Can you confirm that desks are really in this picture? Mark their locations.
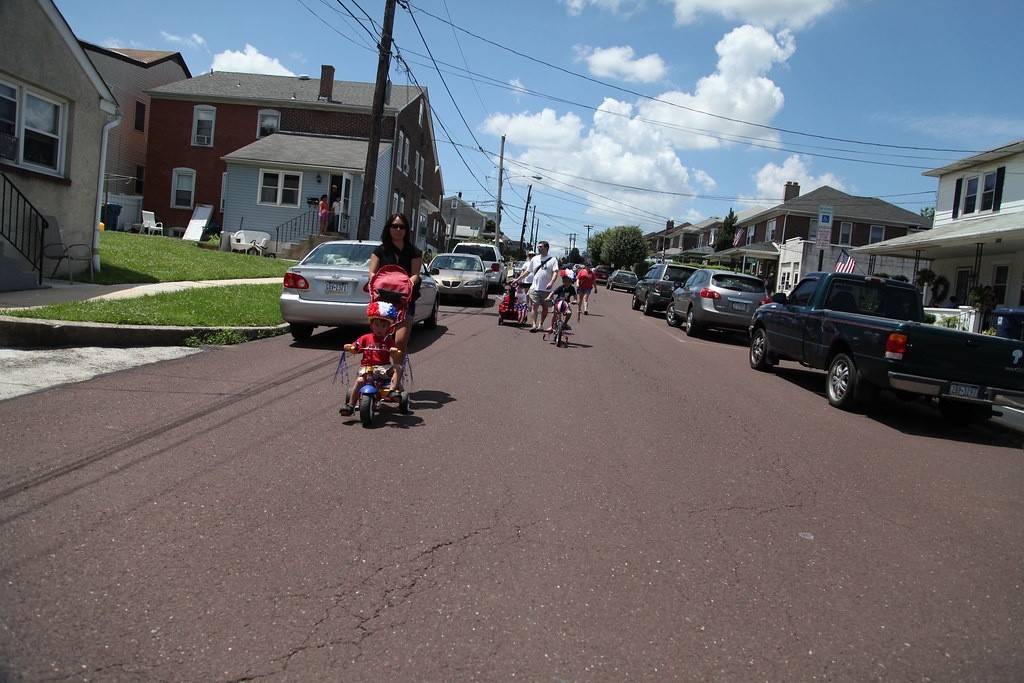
[169,227,187,237]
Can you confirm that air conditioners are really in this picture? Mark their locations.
[194,134,211,145]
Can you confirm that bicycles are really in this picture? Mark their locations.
[543,298,576,347]
[247,240,276,258]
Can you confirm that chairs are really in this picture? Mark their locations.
[830,291,857,314]
[138,210,163,237]
[31,213,94,285]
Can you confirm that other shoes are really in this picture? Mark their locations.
[339,403,355,414]
[584,310,588,315]
[529,326,537,333]
[538,322,544,331]
[385,390,402,403]
[562,324,571,330]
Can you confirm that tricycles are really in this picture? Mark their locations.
[342,266,411,423]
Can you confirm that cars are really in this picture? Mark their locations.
[279,240,440,341]
[424,253,492,305]
[606,270,638,294]
[562,263,579,272]
[513,261,525,278]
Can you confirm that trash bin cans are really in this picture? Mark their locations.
[101,202,122,231]
[992,307,1024,341]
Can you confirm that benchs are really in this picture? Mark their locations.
[230,229,271,256]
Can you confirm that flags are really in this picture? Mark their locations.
[833,250,856,274]
[731,225,744,247]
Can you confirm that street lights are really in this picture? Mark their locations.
[494,175,542,248]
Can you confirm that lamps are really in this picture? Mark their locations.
[316,174,321,181]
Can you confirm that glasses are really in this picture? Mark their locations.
[537,246,543,248]
[392,223,409,229]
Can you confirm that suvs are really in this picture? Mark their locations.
[632,262,698,315]
[592,265,612,283]
[452,243,507,290]
[664,268,767,337]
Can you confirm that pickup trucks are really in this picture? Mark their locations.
[748,270,1024,423]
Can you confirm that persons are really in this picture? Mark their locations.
[369,213,422,390]
[945,296,957,309]
[318,184,341,235]
[543,269,578,332]
[513,284,527,323]
[519,250,537,302]
[336,301,402,416]
[575,261,598,314]
[510,241,559,333]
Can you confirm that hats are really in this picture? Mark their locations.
[366,300,398,326]
[560,268,577,284]
